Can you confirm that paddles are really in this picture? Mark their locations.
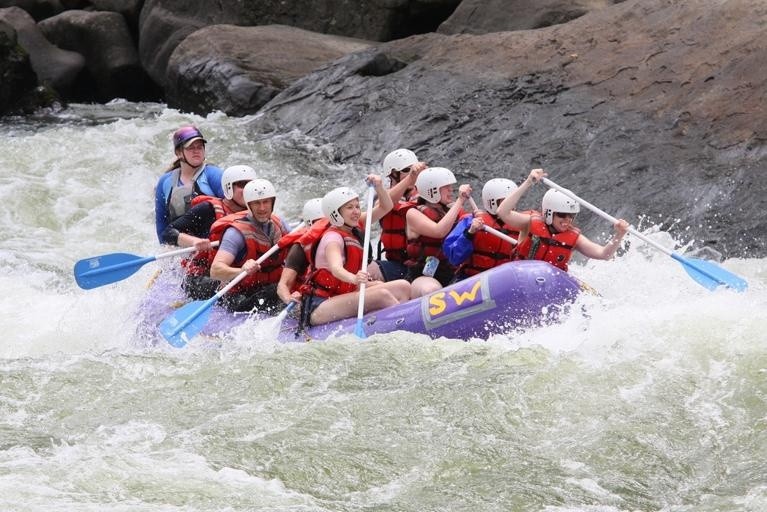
[533,174,749,294]
[73,239,220,291]
[354,180,374,338]
[162,218,305,349]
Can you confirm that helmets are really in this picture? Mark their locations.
[542,187,580,226]
[173,125,204,148]
[383,149,419,177]
[482,178,519,215]
[415,166,458,204]
[221,164,276,217]
[303,186,360,228]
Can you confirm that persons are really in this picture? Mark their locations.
[405,163,471,288]
[444,178,529,287]
[371,148,427,278]
[160,166,255,298]
[275,197,324,304]
[208,178,291,314]
[152,125,225,243]
[298,174,410,328]
[497,168,632,272]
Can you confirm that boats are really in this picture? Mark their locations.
[133,222,607,369]
